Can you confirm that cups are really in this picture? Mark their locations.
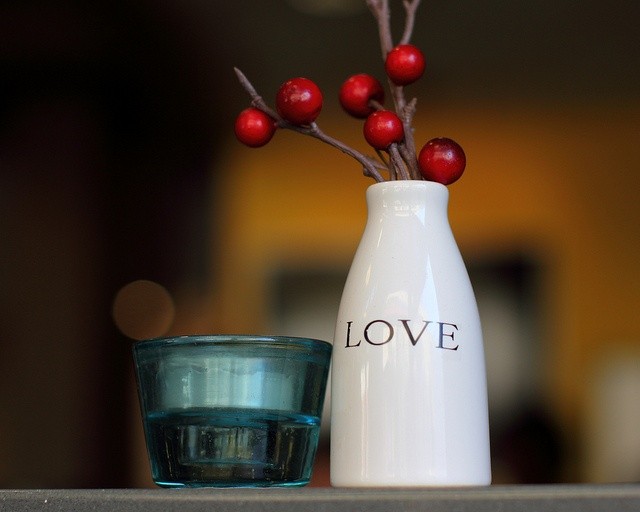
[135,336,332,486]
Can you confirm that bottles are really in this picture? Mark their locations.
[330,178,492,484]
